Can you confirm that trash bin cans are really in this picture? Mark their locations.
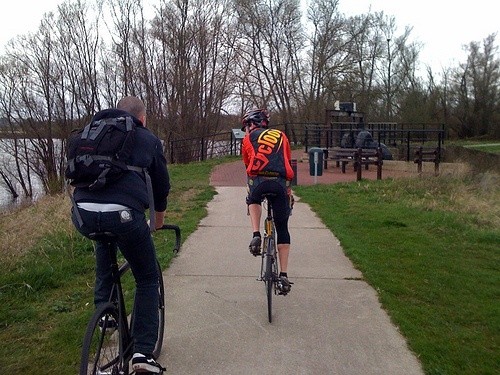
[308,148,324,177]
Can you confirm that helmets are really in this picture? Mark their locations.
[241,108,269,131]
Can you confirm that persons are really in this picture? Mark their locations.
[241,108,295,288]
[66,96,171,375]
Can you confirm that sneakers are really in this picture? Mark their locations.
[99,318,118,333]
[132,353,162,374]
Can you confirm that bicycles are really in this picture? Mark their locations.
[79,224,182,375]
[246,193,295,322]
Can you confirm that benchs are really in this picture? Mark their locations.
[324,148,377,172]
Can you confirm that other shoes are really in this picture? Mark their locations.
[279,276,290,293]
[250,237,260,255]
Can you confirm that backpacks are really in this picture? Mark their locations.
[68,116,148,191]
